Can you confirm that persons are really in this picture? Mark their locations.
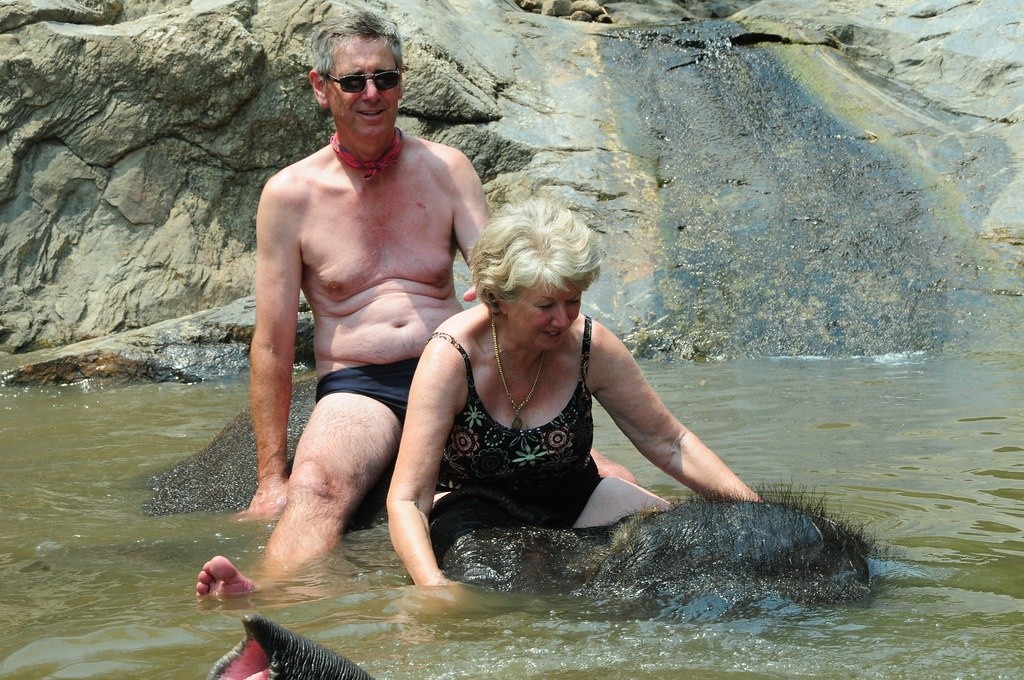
[386,196,766,590]
[193,11,493,606]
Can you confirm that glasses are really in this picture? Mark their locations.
[321,67,400,93]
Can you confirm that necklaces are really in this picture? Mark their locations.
[491,314,545,430]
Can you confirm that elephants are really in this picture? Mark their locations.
[141,377,897,680]
